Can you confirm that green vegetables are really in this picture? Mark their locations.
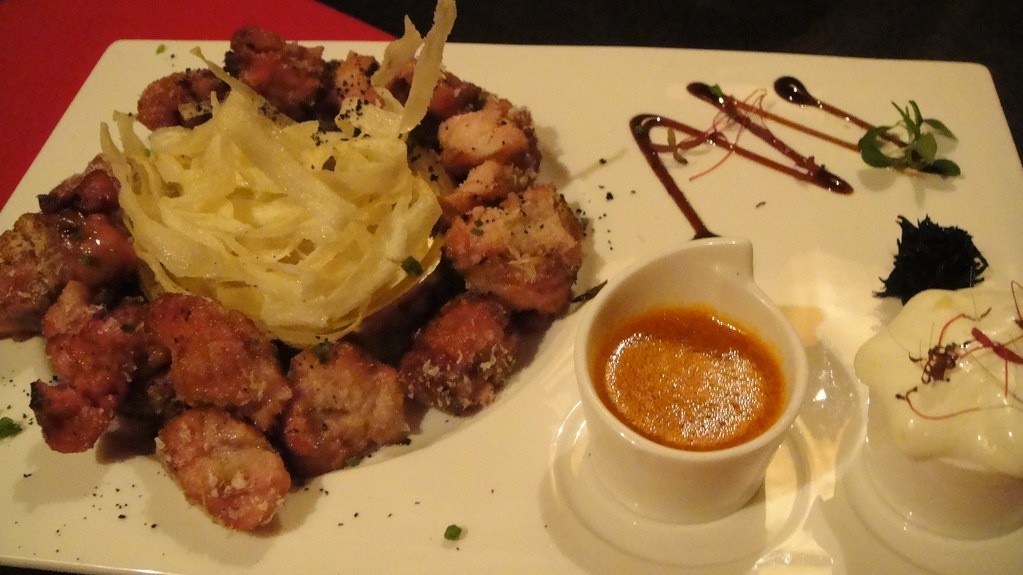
[859,97,962,178]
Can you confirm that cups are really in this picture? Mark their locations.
[862,393,1023,540]
[577,238,811,526]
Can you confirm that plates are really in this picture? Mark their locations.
[2,37,1023,574]
[541,399,821,569]
